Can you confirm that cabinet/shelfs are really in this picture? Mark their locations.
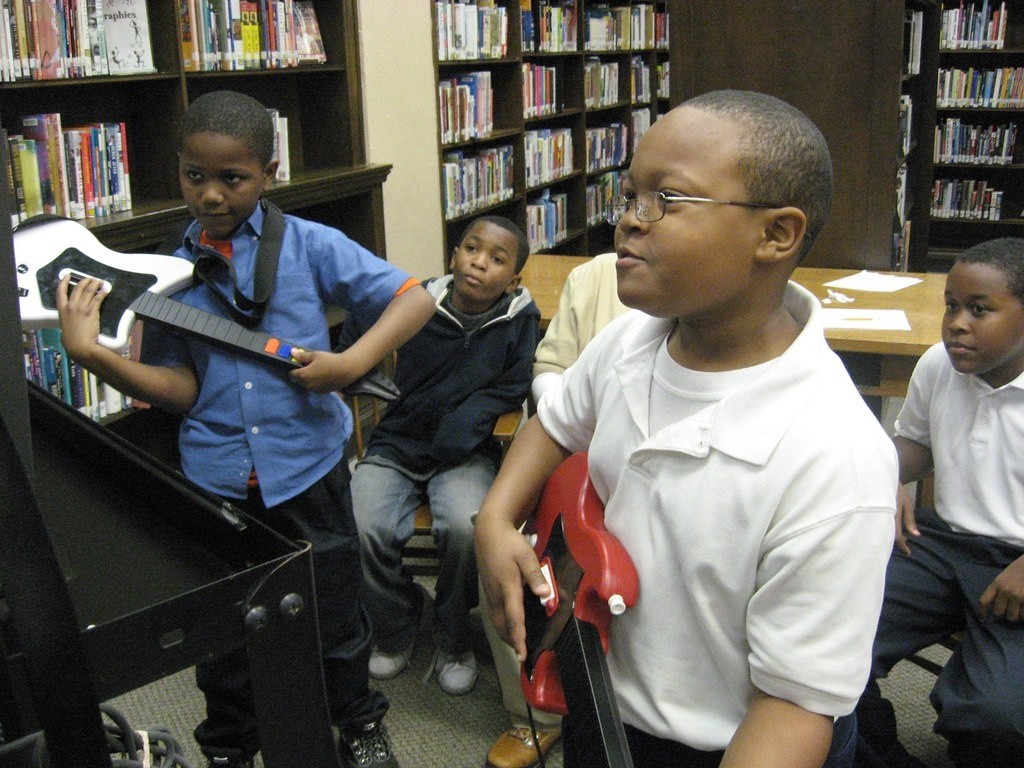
[431,0,674,276]
[892,0,1023,274]
[1,0,393,464]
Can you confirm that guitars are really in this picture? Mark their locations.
[520,451,641,767]
[12,213,400,403]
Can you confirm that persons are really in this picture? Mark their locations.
[473,87,902,767]
[346,214,542,699]
[856,234,1024,766]
[54,89,437,767]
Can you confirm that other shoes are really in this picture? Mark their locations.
[338,721,400,768]
[201,745,254,768]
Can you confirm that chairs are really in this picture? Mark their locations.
[351,391,523,576]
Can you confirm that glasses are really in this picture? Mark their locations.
[601,191,811,239]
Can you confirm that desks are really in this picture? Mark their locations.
[521,253,948,509]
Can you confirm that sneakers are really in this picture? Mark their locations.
[438,650,477,695]
[368,636,414,679]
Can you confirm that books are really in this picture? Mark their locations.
[436,2,670,268]
[0,0,392,429]
[889,2,1024,268]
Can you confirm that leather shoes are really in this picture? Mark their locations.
[487,726,561,768]
